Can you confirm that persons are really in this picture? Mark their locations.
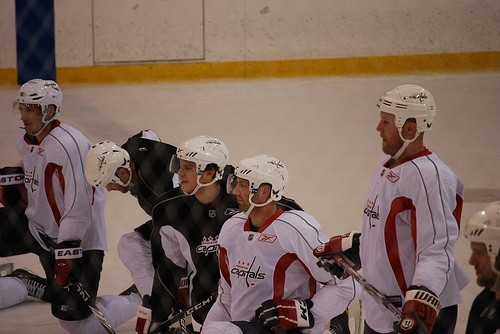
[0,78,141,334]
[464,200,500,334]
[312,82,471,334]
[85,128,364,334]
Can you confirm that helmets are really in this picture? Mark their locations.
[464,201,500,274]
[85,140,132,190]
[12,79,63,123]
[377,84,436,142]
[227,154,289,206]
[169,135,228,186]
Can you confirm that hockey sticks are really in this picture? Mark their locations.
[37,224,117,334]
[178,305,187,334]
[150,292,217,334]
[333,254,402,321]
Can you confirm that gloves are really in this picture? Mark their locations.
[135,295,169,334]
[0,166,28,209]
[313,232,362,280]
[255,299,315,332]
[393,285,441,334]
[51,240,84,292]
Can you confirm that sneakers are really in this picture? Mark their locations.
[12,269,50,302]
[327,298,362,334]
